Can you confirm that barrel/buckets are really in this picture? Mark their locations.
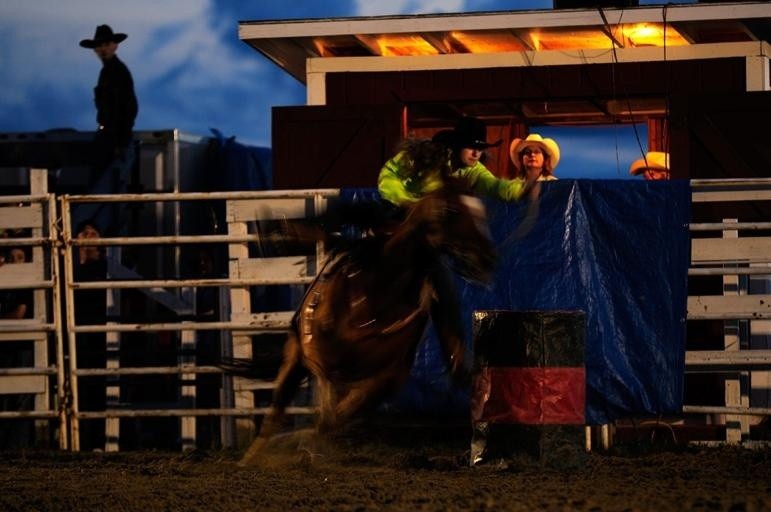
[472,308,587,471]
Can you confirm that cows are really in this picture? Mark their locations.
[198,163,497,467]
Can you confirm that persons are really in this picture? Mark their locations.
[254,117,541,371]
[0,248,28,319]
[73,222,103,281]
[78,24,139,193]
[506,131,560,181]
[629,150,670,180]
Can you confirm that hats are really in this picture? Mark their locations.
[440,117,503,150]
[630,151,672,177]
[80,24,127,48]
[509,133,560,173]
